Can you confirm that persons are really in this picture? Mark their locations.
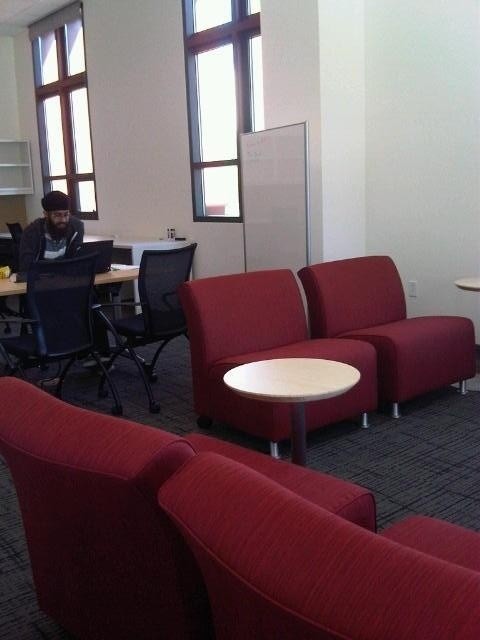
[18,191,114,376]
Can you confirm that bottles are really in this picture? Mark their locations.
[167,228,175,241]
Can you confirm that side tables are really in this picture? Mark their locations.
[224,357,361,467]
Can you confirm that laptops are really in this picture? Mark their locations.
[76,240,113,275]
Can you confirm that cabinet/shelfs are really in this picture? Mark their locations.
[0,140,36,195]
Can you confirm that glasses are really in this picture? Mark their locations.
[52,213,70,218]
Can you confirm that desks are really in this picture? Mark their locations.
[0,261,180,366]
[0,232,190,317]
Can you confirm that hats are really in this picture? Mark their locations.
[41,191,73,210]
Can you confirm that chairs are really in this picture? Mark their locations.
[0,251,123,416]
[180,268,378,458]
[92,243,197,412]
[0,374,377,640]
[5,220,23,244]
[297,255,476,419]
[155,450,478,639]
